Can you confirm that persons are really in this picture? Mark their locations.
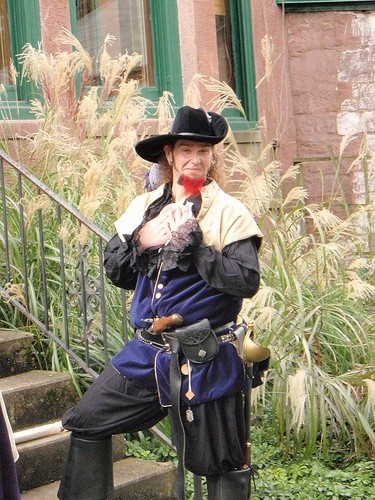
[57,106,270,500]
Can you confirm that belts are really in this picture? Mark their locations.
[138,326,236,500]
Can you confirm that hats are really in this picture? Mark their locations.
[135,106,228,163]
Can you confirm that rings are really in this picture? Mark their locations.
[171,231,176,235]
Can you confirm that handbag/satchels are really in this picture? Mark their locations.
[175,318,219,363]
